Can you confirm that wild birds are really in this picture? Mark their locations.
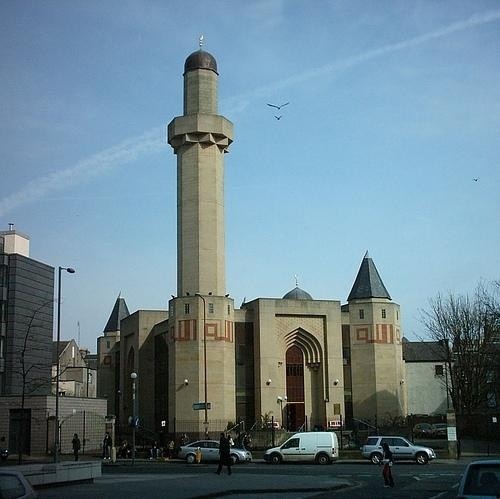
[274,116,281,120]
[267,102,290,110]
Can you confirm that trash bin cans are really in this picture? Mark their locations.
[147,448,164,457]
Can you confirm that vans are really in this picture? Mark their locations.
[263,432,339,465]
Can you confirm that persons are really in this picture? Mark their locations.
[152,433,190,459]
[228,433,253,453]
[214,433,232,476]
[196,446,203,464]
[381,442,395,488]
[72,434,80,461]
[119,439,132,462]
[103,432,112,459]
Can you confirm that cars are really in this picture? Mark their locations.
[413,423,446,439]
[266,421,281,431]
[457,457,500,499]
[0,471,36,499]
[178,440,252,464]
[360,436,436,465]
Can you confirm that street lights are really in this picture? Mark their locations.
[195,293,209,446]
[130,372,137,466]
[54,267,75,463]
[487,416,497,453]
